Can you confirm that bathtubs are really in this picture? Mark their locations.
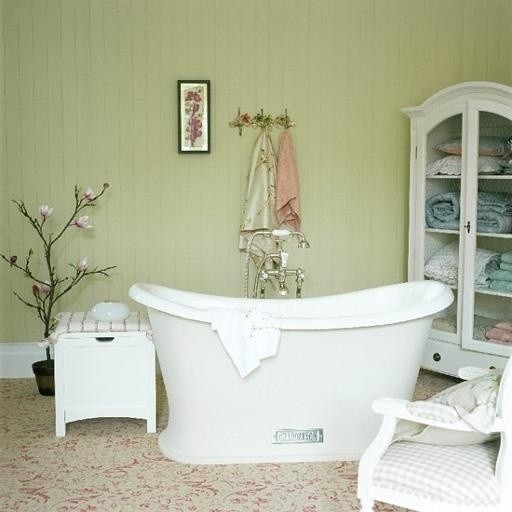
[129,279,455,466]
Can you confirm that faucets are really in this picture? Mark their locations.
[277,272,288,295]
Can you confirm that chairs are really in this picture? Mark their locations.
[357,355,512,512]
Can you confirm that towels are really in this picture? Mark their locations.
[276,129,302,234]
[484,319,512,347]
[239,128,276,259]
[488,252,512,294]
[208,305,281,379]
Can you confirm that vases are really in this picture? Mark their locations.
[31,358,58,397]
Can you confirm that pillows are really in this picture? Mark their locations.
[432,136,512,159]
[388,368,504,446]
[426,154,505,176]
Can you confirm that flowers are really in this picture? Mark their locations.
[184,89,204,146]
[0,180,118,366]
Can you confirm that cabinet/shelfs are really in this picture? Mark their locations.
[400,80,512,379]
[44,312,160,438]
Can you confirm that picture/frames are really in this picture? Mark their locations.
[177,80,210,154]
